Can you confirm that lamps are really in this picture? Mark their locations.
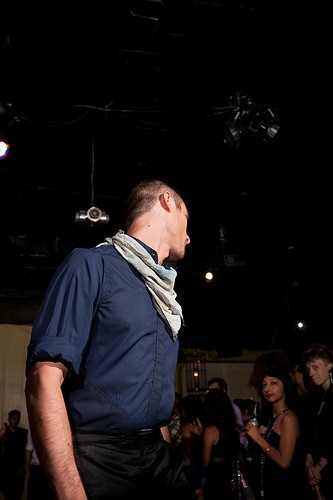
[213,91,281,152]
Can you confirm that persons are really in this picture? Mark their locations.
[24,429,35,479]
[159,342,333,500]
[25,176,200,500]
[0,410,29,500]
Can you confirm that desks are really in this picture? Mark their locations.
[175,360,263,405]
